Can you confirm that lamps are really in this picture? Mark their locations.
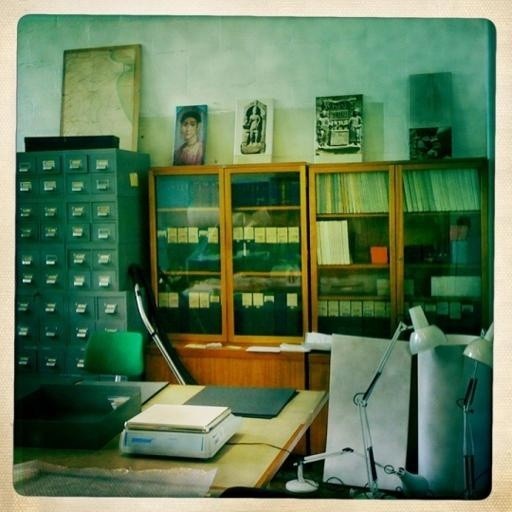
[285,306,493,497]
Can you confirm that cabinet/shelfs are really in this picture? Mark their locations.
[308,158,489,453]
[16,150,151,370]
[148,162,310,455]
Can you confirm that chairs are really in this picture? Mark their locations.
[84,330,145,382]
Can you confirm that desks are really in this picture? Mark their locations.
[14,384,329,498]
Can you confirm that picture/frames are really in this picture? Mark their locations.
[61,46,139,151]
[313,94,358,158]
[233,98,273,164]
[176,106,205,162]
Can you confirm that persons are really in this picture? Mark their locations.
[246,106,261,146]
[173,112,202,164]
[317,105,361,146]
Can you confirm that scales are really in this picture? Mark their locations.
[119,404,240,461]
